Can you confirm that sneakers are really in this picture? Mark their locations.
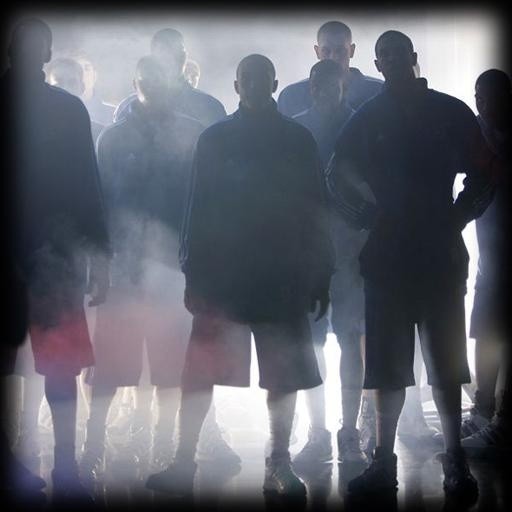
[2,403,241,495]
[293,425,334,464]
[458,407,489,436]
[358,412,378,455]
[348,454,398,495]
[262,455,306,498]
[459,422,508,451]
[336,428,369,465]
[436,448,479,504]
[397,417,439,438]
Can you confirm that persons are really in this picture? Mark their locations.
[1,18,512,499]
